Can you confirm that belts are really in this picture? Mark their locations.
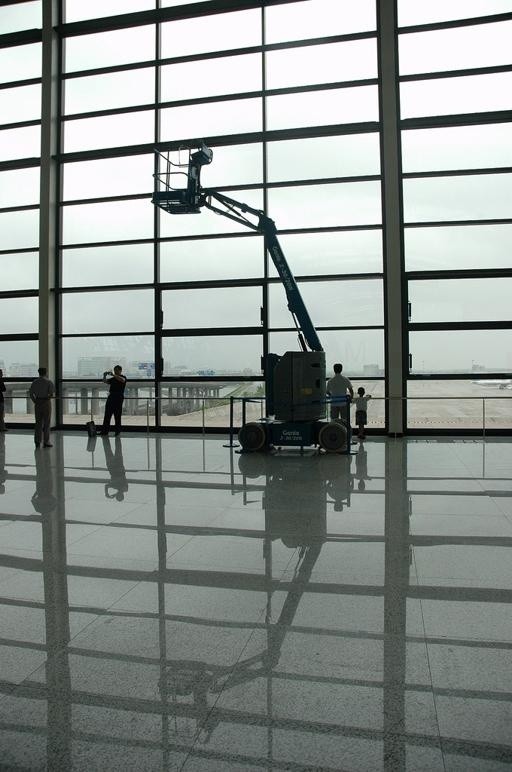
[36,398,50,400]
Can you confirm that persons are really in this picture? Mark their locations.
[326,364,357,444]
[97,365,127,435]
[348,387,371,439]
[30,367,54,447]
[0,369,8,432]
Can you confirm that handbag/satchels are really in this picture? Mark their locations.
[86,421,96,437]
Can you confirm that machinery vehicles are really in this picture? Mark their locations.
[154,136,360,458]
[148,454,355,743]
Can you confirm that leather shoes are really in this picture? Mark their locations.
[96,430,120,436]
[35,442,53,447]
